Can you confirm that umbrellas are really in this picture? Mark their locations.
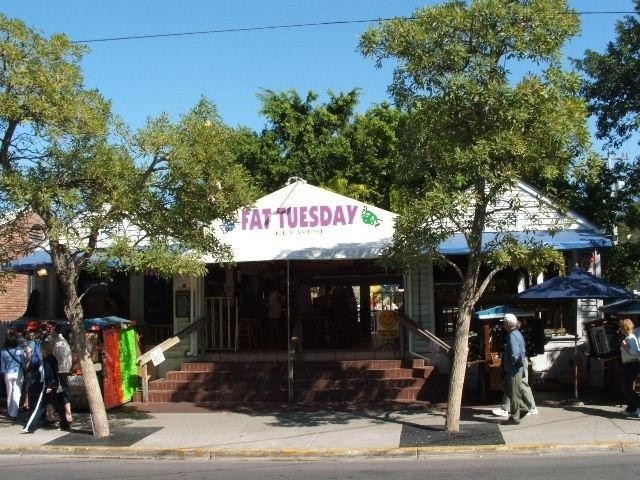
[514,265,637,399]
[597,290,640,319]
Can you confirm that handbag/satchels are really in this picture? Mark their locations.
[515,357,529,367]
[619,347,640,363]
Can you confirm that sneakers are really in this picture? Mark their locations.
[491,406,538,425]
[622,409,640,418]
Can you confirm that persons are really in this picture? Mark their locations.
[0,319,73,433]
[490,319,539,418]
[498,311,526,424]
[618,317,640,418]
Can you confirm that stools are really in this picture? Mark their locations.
[239,319,264,346]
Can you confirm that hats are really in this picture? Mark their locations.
[29,322,37,329]
[500,313,516,323]
